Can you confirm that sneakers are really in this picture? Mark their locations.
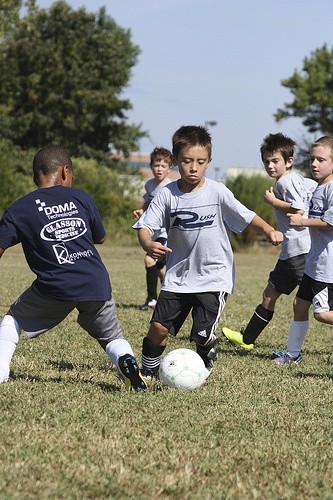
[139,295,158,311]
[272,353,303,365]
[141,372,157,385]
[221,326,254,351]
[117,353,148,393]
[202,358,214,379]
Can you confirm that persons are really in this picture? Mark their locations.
[131,125,284,380]
[133,146,173,310]
[221,133,319,349]
[0,145,148,393]
[271,136,333,365]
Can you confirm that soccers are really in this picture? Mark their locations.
[157,348,209,389]
